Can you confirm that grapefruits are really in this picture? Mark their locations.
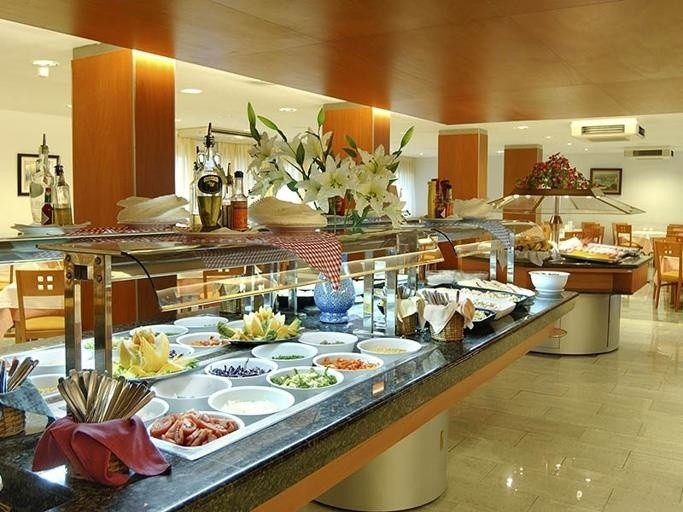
[119,332,169,372]
[243,310,285,336]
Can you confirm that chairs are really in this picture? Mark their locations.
[15,270,64,343]
[563,221,683,312]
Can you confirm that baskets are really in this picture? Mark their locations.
[423,293,466,342]
[64,441,130,480]
[379,287,418,335]
[0,403,26,439]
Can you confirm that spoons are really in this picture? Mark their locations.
[57,368,156,423]
[382,283,418,298]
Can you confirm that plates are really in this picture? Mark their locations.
[263,222,326,232]
[182,231,261,245]
[423,217,464,223]
[117,220,179,229]
[324,214,352,221]
[13,226,87,235]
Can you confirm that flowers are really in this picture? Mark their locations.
[522,151,589,190]
[246,101,415,235]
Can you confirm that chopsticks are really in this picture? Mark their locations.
[422,289,461,306]
[1,356,39,392]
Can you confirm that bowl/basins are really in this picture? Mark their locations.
[296,331,358,355]
[223,319,246,331]
[357,337,423,364]
[251,342,319,368]
[203,357,278,385]
[265,366,344,400]
[174,333,230,352]
[174,316,228,333]
[313,352,384,379]
[167,342,196,358]
[120,366,192,382]
[229,331,300,348]
[150,373,232,414]
[147,411,246,447]
[528,271,571,295]
[134,397,169,425]
[128,324,190,342]
[208,386,296,425]
[528,295,564,315]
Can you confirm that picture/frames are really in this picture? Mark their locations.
[589,167,623,195]
[17,153,59,196]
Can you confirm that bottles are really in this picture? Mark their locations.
[191,122,249,232]
[427,178,454,218]
[29,135,74,225]
[329,196,347,214]
